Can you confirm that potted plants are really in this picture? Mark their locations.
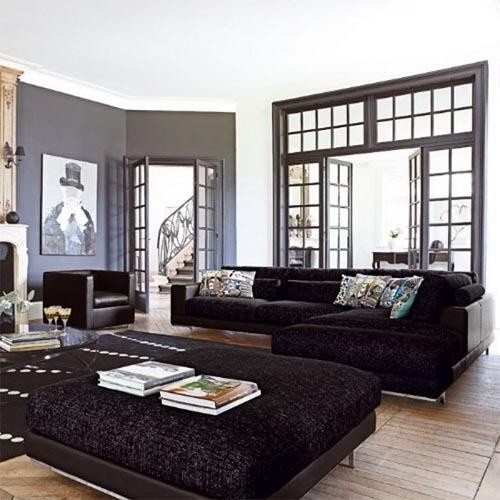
[0,289,35,333]
[288,214,312,248]
[387,228,401,252]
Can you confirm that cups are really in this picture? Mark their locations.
[44,308,55,335]
[59,308,71,336]
[51,306,61,333]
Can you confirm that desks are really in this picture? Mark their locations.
[372,252,455,272]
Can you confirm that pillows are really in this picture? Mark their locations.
[407,273,473,323]
[343,274,392,309]
[333,274,355,305]
[390,278,424,319]
[252,278,282,300]
[286,279,341,302]
[380,278,408,308]
[199,269,221,296]
[216,269,256,299]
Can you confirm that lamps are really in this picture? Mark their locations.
[6,146,26,169]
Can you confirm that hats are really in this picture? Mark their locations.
[60,163,84,191]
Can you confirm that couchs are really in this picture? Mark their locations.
[24,344,383,500]
[43,269,136,330]
[171,265,478,335]
[271,273,496,405]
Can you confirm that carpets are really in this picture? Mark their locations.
[0,330,272,463]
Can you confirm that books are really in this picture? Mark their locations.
[158,374,263,416]
[0,330,62,353]
[95,359,196,397]
